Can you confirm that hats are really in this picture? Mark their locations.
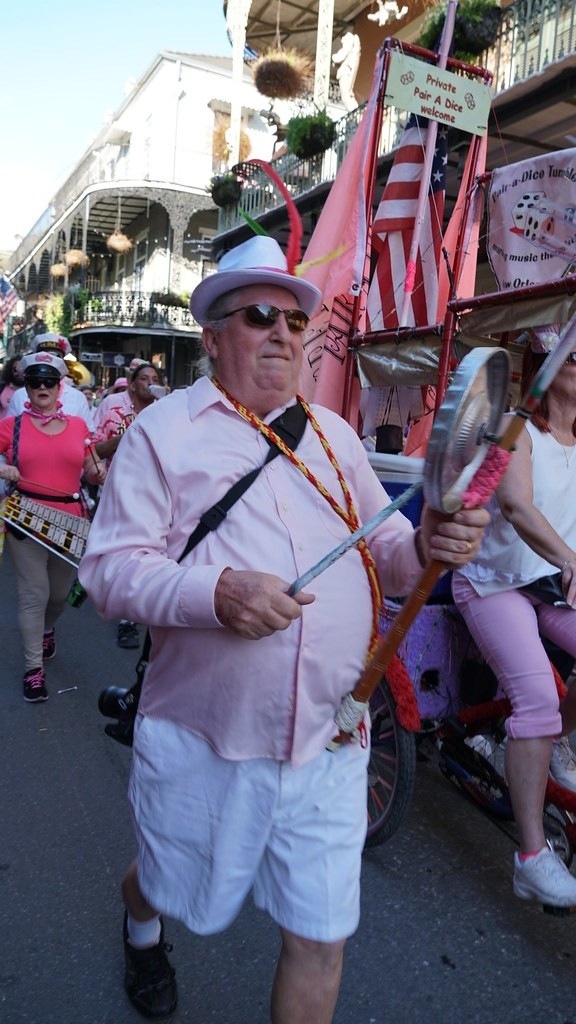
[16,351,69,382]
[190,236,322,325]
[109,377,128,395]
[31,334,72,357]
[124,358,146,374]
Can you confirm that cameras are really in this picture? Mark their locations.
[98,685,139,748]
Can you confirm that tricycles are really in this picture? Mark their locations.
[352,276,575,919]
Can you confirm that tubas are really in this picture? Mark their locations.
[63,360,91,388]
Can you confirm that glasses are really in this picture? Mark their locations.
[563,352,576,367]
[13,367,17,372]
[27,377,59,389]
[217,304,309,331]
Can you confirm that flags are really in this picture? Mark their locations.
[287,37,489,459]
[0,277,20,326]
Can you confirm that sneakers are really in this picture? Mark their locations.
[117,621,141,648]
[513,838,576,906]
[43,627,57,660]
[22,667,49,701]
[123,912,179,1020]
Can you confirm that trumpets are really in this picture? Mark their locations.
[116,404,137,436]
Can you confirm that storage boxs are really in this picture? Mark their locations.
[367,451,453,599]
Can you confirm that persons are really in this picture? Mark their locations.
[95,358,171,650]
[331,32,361,123]
[0,332,107,703]
[451,323,576,909]
[76,236,490,1023]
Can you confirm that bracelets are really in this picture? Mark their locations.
[560,557,576,572]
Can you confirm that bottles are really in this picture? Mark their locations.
[148,384,166,400]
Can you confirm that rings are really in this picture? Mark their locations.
[466,542,472,553]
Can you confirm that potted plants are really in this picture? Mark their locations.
[284,105,338,160]
[41,286,90,341]
[151,286,190,309]
[414,0,507,63]
[205,172,243,207]
